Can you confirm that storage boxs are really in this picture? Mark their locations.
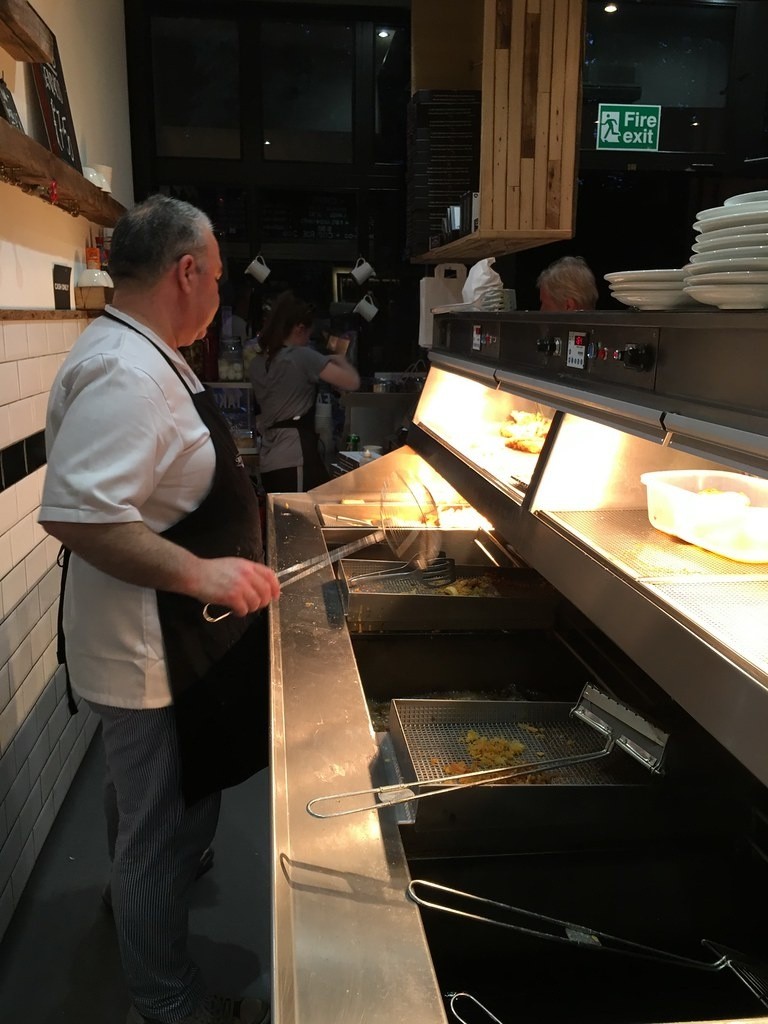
[640,469,768,565]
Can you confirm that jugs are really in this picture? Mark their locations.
[350,257,377,286]
[352,294,379,322]
[244,255,271,283]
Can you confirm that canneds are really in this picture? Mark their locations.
[345,435,359,451]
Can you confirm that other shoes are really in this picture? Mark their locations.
[100,847,215,908]
[144,992,270,1024]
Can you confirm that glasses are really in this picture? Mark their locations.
[296,303,318,326]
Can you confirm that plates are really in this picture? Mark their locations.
[603,269,695,310]
[683,190,768,310]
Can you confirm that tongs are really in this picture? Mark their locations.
[429,289,516,313]
[348,550,457,587]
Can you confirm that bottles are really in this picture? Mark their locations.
[371,375,427,393]
[88,227,111,273]
[218,335,245,382]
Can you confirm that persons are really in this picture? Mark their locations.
[250,290,361,493]
[37,196,279,1022]
